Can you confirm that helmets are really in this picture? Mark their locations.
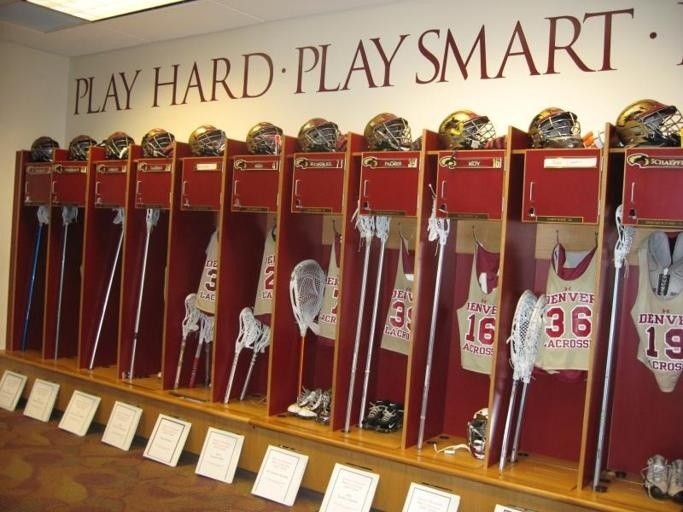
[67,136,98,160]
[438,109,497,150]
[246,122,283,154]
[364,113,416,152]
[616,99,683,148]
[467,407,488,460]
[142,128,175,157]
[105,132,135,159]
[297,118,344,152]
[189,125,225,155]
[528,107,584,148]
[30,137,58,162]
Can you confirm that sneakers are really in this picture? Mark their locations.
[287,385,332,426]
[362,400,404,433]
[640,455,682,505]
